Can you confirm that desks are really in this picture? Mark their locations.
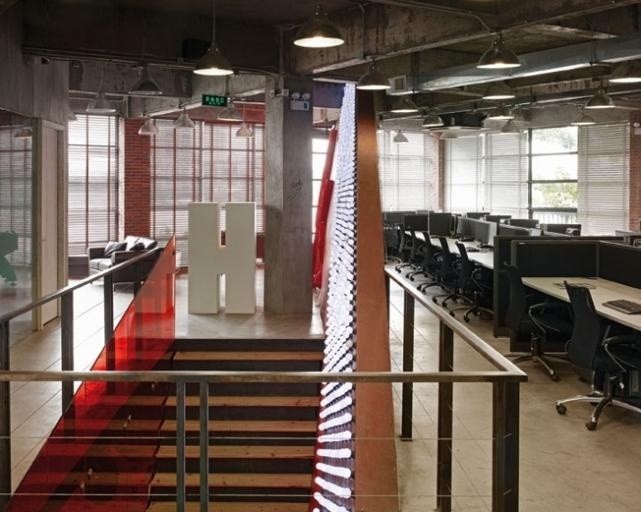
[523,276,641,383]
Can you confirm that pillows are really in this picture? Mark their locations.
[127,241,145,253]
[102,238,124,261]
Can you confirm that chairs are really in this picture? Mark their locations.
[555,279,641,431]
[502,261,576,381]
[382,209,497,325]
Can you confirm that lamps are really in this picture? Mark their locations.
[291,2,346,50]
[472,30,526,70]
[191,0,235,79]
[355,55,391,90]
[137,101,254,139]
[481,79,516,103]
[126,62,168,97]
[85,63,120,115]
[584,28,617,111]
[392,106,523,143]
[569,108,597,126]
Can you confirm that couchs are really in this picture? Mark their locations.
[86,232,164,291]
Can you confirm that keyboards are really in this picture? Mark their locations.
[602,299,641,314]
